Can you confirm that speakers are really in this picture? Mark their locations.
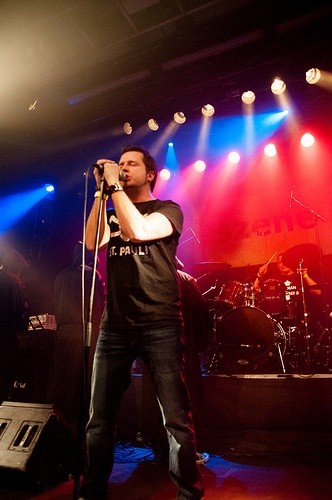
[0,401,87,492]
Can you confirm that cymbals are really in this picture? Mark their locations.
[194,261,232,272]
[280,243,323,270]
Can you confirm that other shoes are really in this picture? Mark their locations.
[196,452,210,464]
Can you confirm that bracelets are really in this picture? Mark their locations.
[106,179,124,195]
[91,191,110,200]
[301,272,309,279]
[93,184,110,194]
[257,274,264,281]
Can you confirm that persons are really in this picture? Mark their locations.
[80,140,205,500]
[53,241,104,413]
[1,249,32,332]
[252,248,322,336]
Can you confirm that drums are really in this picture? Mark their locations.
[214,306,287,373]
[215,280,243,308]
[260,279,285,315]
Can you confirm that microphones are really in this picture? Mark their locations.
[290,192,293,208]
[93,163,126,182]
[189,228,201,244]
